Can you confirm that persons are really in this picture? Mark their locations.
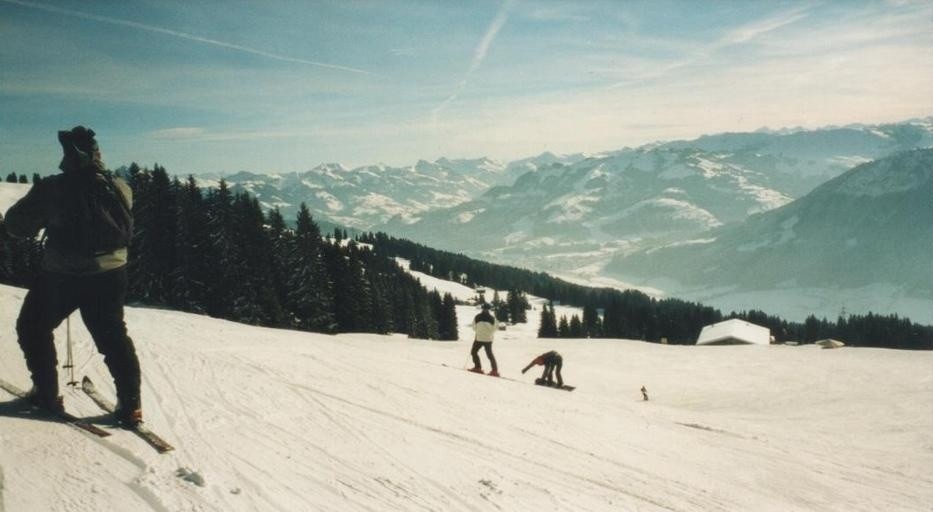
[522,351,563,387]
[471,305,498,377]
[4,125,141,427]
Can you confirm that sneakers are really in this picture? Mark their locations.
[113,404,144,427]
[8,390,65,414]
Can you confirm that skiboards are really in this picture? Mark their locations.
[0,375,176,454]
[441,364,525,384]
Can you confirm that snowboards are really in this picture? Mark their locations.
[535,378,576,391]
